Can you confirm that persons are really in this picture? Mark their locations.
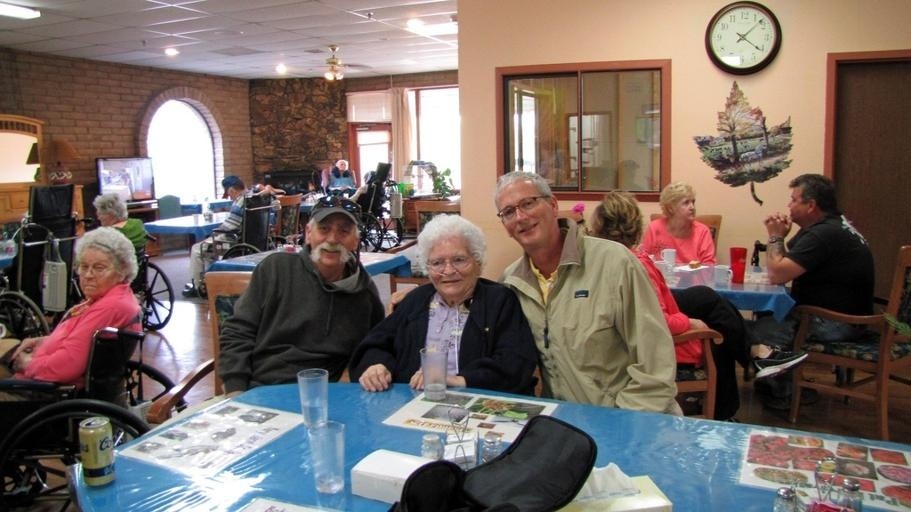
[211,194,386,399]
[752,172,878,418]
[348,213,538,397]
[348,170,379,205]
[327,159,358,198]
[591,186,808,425]
[635,179,717,267]
[0,224,144,401]
[105,164,149,192]
[670,262,715,291]
[493,168,685,418]
[180,175,255,299]
[91,191,149,247]
[536,138,656,190]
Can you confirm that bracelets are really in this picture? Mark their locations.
[767,235,785,243]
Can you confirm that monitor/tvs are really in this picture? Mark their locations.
[95,157,158,208]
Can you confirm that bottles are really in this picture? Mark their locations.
[771,487,796,512]
[421,434,442,458]
[480,432,502,462]
[835,478,864,511]
[201,196,214,221]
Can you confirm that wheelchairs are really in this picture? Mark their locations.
[0,304,185,509]
[200,192,276,301]
[327,161,406,256]
[0,181,94,341]
[69,229,176,334]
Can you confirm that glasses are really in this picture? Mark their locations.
[496,195,550,218]
[318,196,360,213]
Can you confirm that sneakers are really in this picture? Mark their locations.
[766,385,818,409]
[183,283,207,297]
[752,347,808,379]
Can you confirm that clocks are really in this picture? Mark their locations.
[706,0,782,76]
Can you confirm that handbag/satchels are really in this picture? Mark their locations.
[42,240,67,312]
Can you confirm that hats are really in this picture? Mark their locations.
[310,196,360,225]
[221,176,240,199]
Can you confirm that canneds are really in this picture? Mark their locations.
[78,418,113,487]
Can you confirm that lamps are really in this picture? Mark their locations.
[26,143,41,181]
[41,141,81,185]
[322,44,344,82]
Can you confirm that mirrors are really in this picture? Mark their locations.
[0,114,45,183]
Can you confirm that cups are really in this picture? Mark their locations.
[304,192,324,203]
[730,247,747,284]
[420,345,449,401]
[713,264,733,284]
[297,368,329,429]
[308,421,344,493]
[343,193,349,198]
[661,248,676,266]
[654,261,667,271]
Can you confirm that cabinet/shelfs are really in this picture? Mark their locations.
[0,184,84,236]
